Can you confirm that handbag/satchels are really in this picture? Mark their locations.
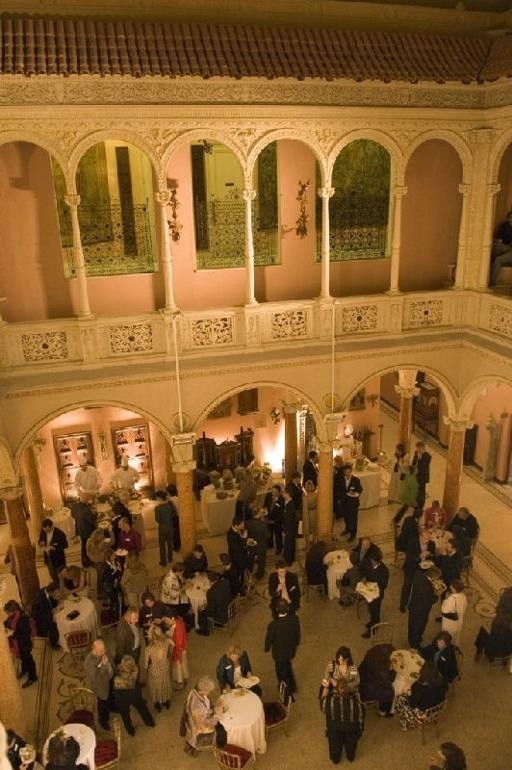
[319,672,334,699]
[441,613,458,621]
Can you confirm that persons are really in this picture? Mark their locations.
[1,431,512,770]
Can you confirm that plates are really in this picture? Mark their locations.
[248,675,260,685]
[247,541,258,546]
[21,749,37,764]
[346,492,359,497]
[115,548,128,557]
[418,560,434,570]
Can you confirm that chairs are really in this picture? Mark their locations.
[94,716,122,770]
[58,567,98,600]
[448,646,465,699]
[457,555,472,588]
[64,630,92,657]
[406,700,447,746]
[263,680,291,740]
[299,563,326,603]
[345,585,370,618]
[370,622,395,648]
[180,709,216,755]
[213,743,255,770]
[210,592,239,639]
[357,686,381,717]
[101,593,123,634]
[66,687,97,727]
[393,524,406,565]
[240,569,252,613]
[468,523,480,555]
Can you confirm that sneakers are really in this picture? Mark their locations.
[17,670,25,679]
[22,678,37,687]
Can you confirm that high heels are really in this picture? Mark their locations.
[163,702,170,709]
[155,704,161,713]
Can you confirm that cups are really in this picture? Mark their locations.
[350,487,354,495]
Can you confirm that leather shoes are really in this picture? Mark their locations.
[347,536,355,542]
[340,531,348,536]
[379,713,394,719]
[196,629,209,636]
[101,722,110,731]
[366,622,373,627]
[362,629,375,638]
[435,617,442,622]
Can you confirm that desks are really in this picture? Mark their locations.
[182,570,217,629]
[214,688,268,753]
[52,597,102,652]
[426,529,458,557]
[200,478,280,537]
[389,650,426,713]
[42,723,96,770]
[352,464,381,510]
[324,550,353,599]
[42,503,75,541]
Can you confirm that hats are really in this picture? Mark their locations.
[345,424,353,435]
[121,455,128,466]
[79,459,87,465]
